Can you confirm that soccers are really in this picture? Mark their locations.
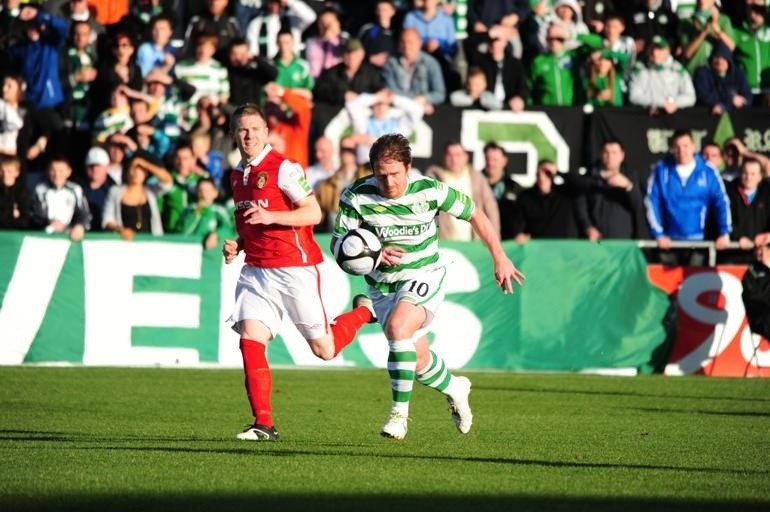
[334,228,384,275]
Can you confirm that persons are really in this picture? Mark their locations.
[1,1,770,240]
[330,132,526,441]
[741,232,770,343]
[221,103,379,442]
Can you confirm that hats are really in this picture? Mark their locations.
[86,147,110,166]
[344,38,361,50]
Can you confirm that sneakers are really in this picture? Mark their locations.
[381,413,408,440]
[446,377,472,434]
[235,423,280,441]
[353,294,379,323]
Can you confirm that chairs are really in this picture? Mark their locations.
[741,261,770,378]
